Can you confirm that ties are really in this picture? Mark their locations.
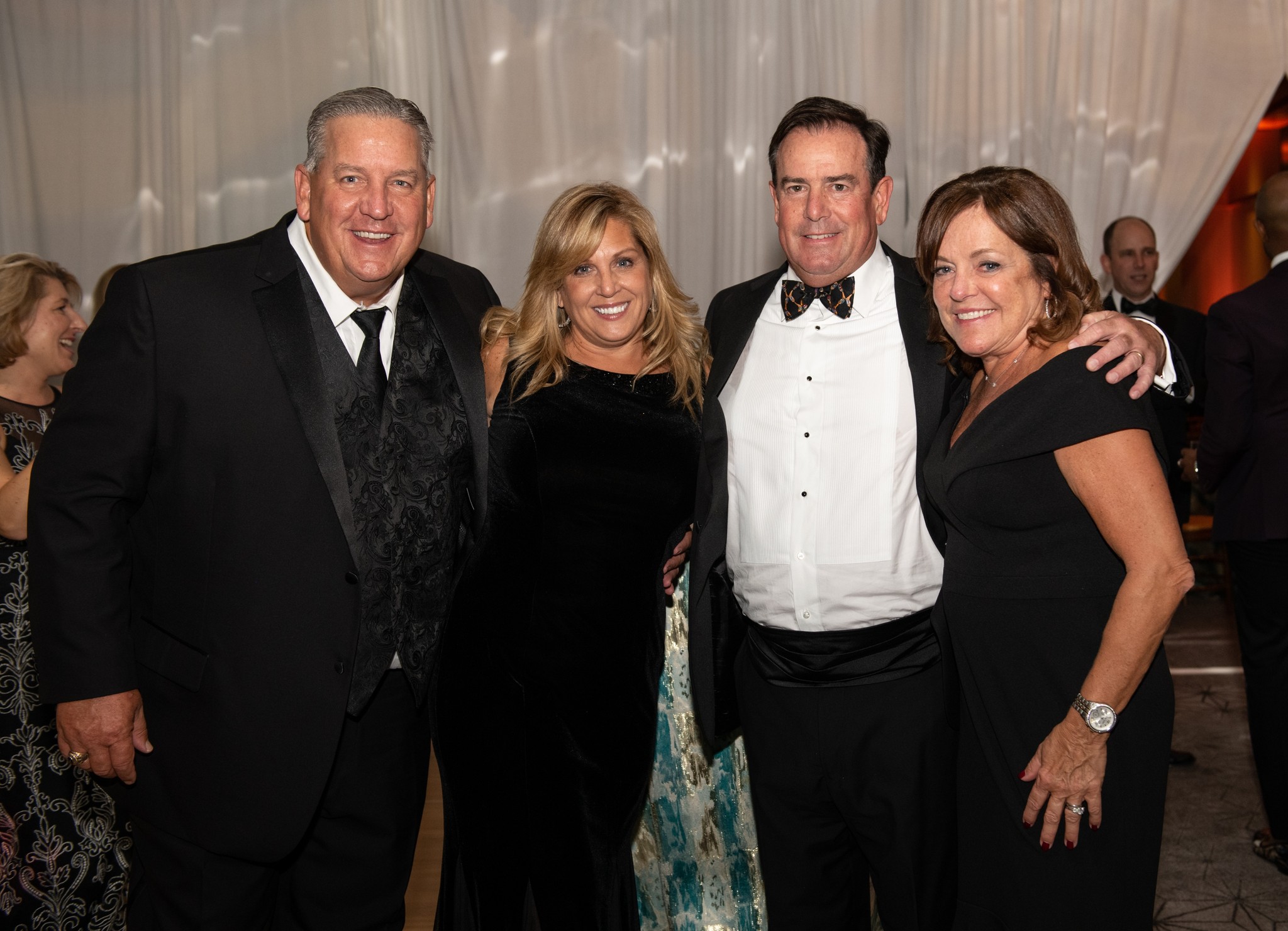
[350,306,391,405]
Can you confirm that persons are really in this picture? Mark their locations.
[916,168,1199,931]
[0,254,134,931]
[685,98,1197,931]
[27,84,695,931]
[430,184,719,930]
[1204,170,1288,877]
[1102,215,1209,553]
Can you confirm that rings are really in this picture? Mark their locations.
[1065,802,1085,815]
[1127,351,1144,367]
[67,750,90,765]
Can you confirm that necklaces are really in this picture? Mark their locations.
[984,342,1031,388]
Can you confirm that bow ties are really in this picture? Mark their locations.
[781,275,856,319]
[1121,297,1156,316]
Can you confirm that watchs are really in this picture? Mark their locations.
[1072,692,1119,734]
